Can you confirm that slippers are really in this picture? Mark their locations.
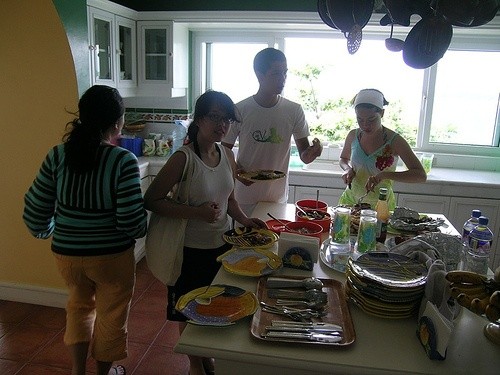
[111,365,126,375]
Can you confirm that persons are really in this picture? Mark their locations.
[143,90,270,375]
[220,47,323,232]
[22,85,149,375]
[337,89,427,211]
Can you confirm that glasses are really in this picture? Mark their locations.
[202,114,234,124]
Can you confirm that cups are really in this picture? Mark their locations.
[353,217,378,261]
[361,210,377,218]
[330,207,352,255]
[420,153,434,175]
[118,132,170,159]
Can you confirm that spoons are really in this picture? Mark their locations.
[267,276,323,289]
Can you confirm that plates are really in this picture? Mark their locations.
[222,226,280,248]
[174,284,260,327]
[319,236,390,274]
[344,250,429,320]
[237,169,286,183]
[265,219,291,235]
[216,248,282,278]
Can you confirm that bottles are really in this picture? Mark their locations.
[466,215,495,276]
[461,210,481,271]
[173,122,186,153]
[375,188,389,244]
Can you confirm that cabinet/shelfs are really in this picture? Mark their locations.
[136,174,500,270]
[86,0,187,99]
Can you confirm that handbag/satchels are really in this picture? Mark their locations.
[144,145,191,286]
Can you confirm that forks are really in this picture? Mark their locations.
[358,183,375,203]
[267,290,328,303]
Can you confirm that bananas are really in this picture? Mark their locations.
[446,265,500,322]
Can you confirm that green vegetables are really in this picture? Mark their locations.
[399,215,430,224]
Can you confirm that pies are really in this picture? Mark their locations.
[251,173,284,179]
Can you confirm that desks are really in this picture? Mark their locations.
[173,201,500,375]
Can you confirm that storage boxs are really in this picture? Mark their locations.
[118,137,143,157]
[316,141,434,174]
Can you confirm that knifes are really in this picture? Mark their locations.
[260,320,343,343]
[349,183,351,189]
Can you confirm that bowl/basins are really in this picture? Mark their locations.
[125,125,146,132]
[284,198,332,246]
